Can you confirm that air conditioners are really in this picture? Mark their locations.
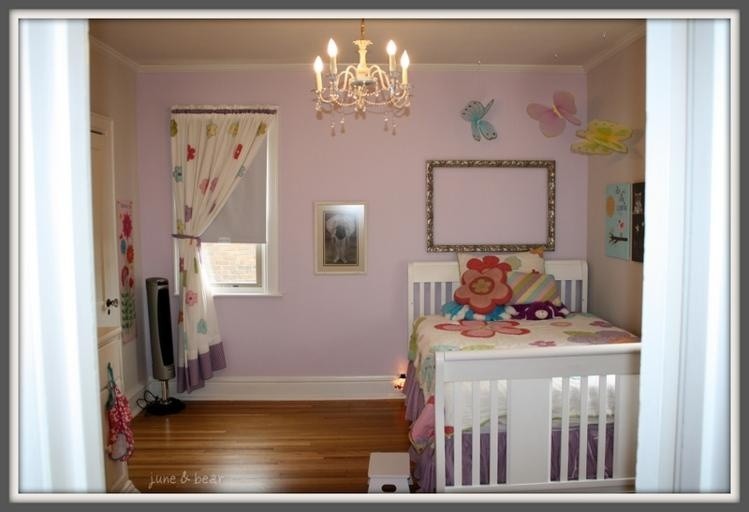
[135,276,187,415]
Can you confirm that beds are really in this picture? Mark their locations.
[406,260,642,495]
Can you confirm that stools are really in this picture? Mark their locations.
[368,451,412,494]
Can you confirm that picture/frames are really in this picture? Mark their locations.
[630,182,648,264]
[312,200,366,275]
[426,159,557,253]
[602,182,631,261]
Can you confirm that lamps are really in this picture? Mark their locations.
[309,20,413,139]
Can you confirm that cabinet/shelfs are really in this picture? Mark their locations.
[94,326,140,496]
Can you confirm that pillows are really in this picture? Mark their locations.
[504,271,562,306]
[459,253,545,285]
[444,299,516,326]
[512,297,566,322]
[456,266,512,313]
